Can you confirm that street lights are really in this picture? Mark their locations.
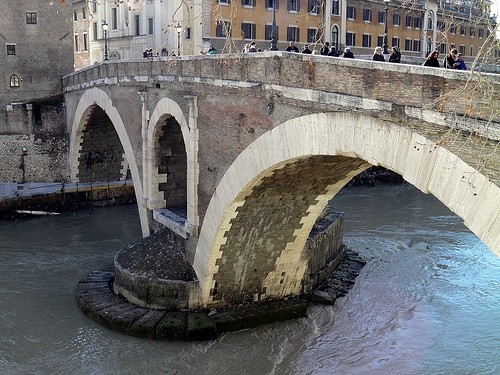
[176,23,182,56]
[101,20,110,60]
[383,0,390,55]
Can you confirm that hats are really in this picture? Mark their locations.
[452,48,457,54]
[394,46,400,52]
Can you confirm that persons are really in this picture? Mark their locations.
[443,48,468,70]
[371,46,385,62]
[328,45,339,57]
[285,40,299,53]
[319,41,330,55]
[342,47,354,58]
[301,44,312,54]
[142,48,154,57]
[241,41,262,53]
[424,50,440,67]
[200,43,218,55]
[389,46,401,63]
